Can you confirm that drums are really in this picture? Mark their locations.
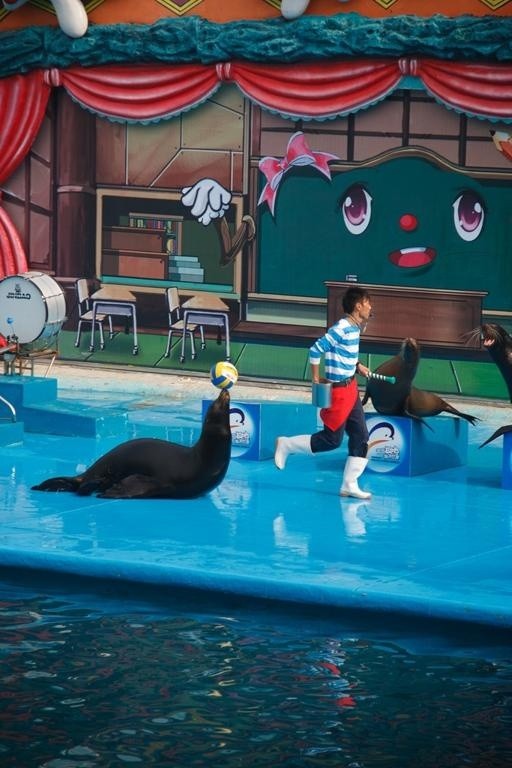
[0,272,66,344]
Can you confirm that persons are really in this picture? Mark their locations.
[272,289,374,500]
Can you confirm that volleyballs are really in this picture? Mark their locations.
[210,361,238,389]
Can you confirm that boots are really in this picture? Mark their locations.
[339,455,371,499]
[275,435,315,470]
